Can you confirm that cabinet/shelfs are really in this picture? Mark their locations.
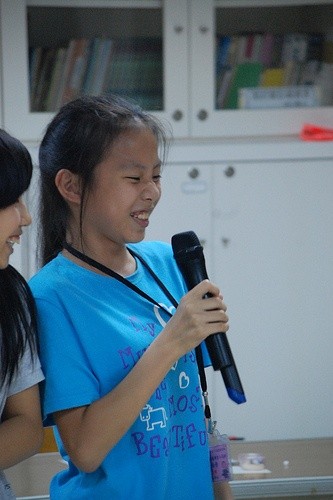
[1,0,333,438]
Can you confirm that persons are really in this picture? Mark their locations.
[0,122,46,499]
[26,92,248,500]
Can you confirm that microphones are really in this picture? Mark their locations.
[171,231,246,404]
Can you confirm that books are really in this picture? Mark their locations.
[31,38,162,110]
[215,34,333,109]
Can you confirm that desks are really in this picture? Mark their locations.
[0,438,332,500]
